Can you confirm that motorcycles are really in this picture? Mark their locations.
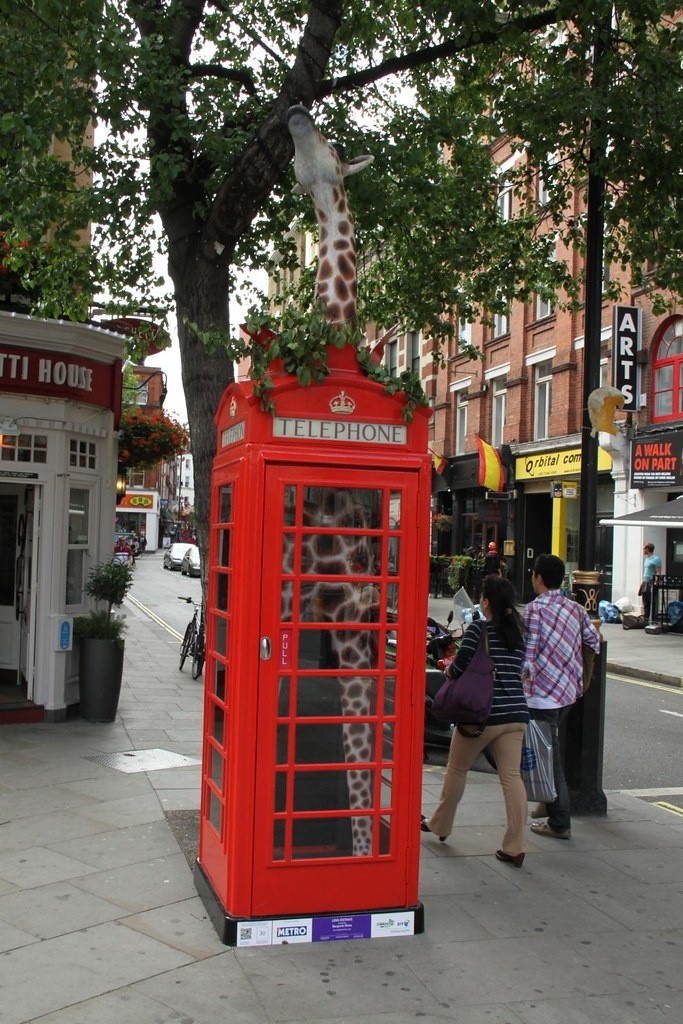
[371,586,498,771]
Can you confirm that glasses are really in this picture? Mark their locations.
[121,539,127,542]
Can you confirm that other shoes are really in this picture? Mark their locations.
[530,805,549,818]
[530,822,572,838]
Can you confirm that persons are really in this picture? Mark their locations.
[520,554,600,839]
[114,530,148,570]
[468,542,510,577]
[421,574,529,868]
[642,544,661,620]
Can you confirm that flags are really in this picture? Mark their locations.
[432,450,447,475]
[475,433,504,494]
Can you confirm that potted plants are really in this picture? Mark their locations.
[72,552,135,723]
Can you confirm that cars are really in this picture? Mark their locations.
[181,546,202,578]
[383,611,462,730]
[162,543,196,571]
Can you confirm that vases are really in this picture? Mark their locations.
[440,528,450,532]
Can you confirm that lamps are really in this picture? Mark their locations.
[116,463,127,504]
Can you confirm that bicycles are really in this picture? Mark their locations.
[178,596,207,680]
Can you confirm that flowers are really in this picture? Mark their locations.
[431,514,457,529]
[118,408,191,480]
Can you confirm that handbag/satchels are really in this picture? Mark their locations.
[520,719,558,804]
[622,610,650,629]
[431,620,497,738]
[598,600,635,624]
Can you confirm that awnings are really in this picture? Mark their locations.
[600,496,683,529]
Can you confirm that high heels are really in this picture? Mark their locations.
[420,814,447,841]
[496,850,525,867]
